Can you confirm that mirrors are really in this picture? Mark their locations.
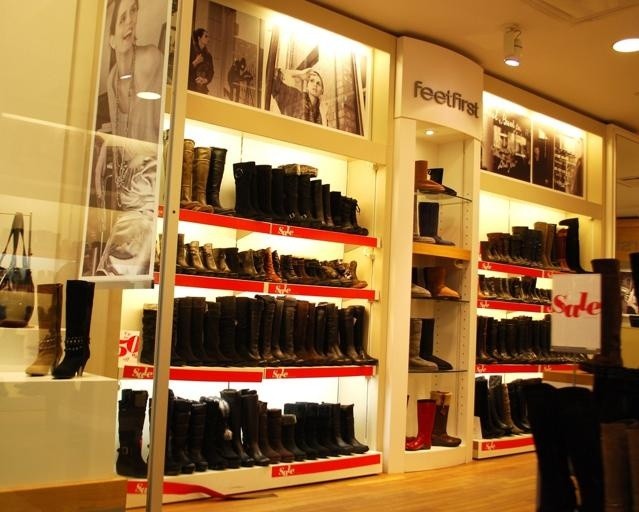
[604,122,639,273]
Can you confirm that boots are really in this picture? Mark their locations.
[327,302,344,365]
[191,402,208,470]
[261,299,280,366]
[419,202,453,244]
[528,229,546,267]
[488,233,508,262]
[310,179,334,229]
[278,296,304,366]
[319,403,339,456]
[205,301,233,365]
[226,247,252,278]
[497,322,512,362]
[220,389,254,468]
[273,250,288,282]
[27,282,64,376]
[416,161,443,192]
[140,304,181,366]
[560,218,589,272]
[531,320,565,362]
[221,398,241,467]
[186,240,211,276]
[422,318,453,371]
[340,402,369,453]
[237,297,267,367]
[504,319,531,362]
[54,278,94,379]
[284,401,316,459]
[511,380,532,430]
[409,317,439,372]
[307,260,326,284]
[199,243,220,276]
[275,298,293,365]
[314,260,339,285]
[406,399,436,451]
[211,248,237,277]
[194,147,213,212]
[270,167,294,224]
[257,401,281,462]
[320,183,341,230]
[216,297,251,366]
[254,164,278,223]
[330,191,350,230]
[159,233,183,272]
[268,409,293,461]
[500,233,521,265]
[295,257,312,282]
[477,315,494,363]
[300,174,322,228]
[117,388,148,478]
[185,297,217,364]
[174,399,195,473]
[344,261,368,288]
[426,267,459,299]
[241,250,264,281]
[310,302,331,365]
[207,147,234,215]
[339,196,363,234]
[331,403,353,454]
[506,324,521,362]
[516,320,537,361]
[264,247,282,282]
[202,396,228,469]
[233,162,271,222]
[479,241,496,261]
[296,401,328,458]
[490,375,513,432]
[282,254,300,283]
[412,281,430,297]
[174,298,202,365]
[339,308,364,364]
[177,234,195,274]
[348,305,378,365]
[148,389,182,475]
[488,316,503,362]
[254,250,271,281]
[241,390,267,465]
[473,378,504,438]
[323,262,353,286]
[281,415,306,461]
[336,306,351,364]
[514,226,536,267]
[536,221,557,269]
[296,299,316,366]
[500,383,523,434]
[430,391,461,447]
[284,172,308,223]
[346,199,369,236]
[181,139,199,209]
[505,237,524,265]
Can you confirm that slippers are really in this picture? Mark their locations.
[426,168,457,196]
[556,226,574,273]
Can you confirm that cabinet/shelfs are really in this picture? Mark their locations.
[0,196,127,512]
[87,1,396,507]
[381,33,485,476]
[474,71,608,461]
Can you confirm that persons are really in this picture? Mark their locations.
[274,68,324,126]
[225,57,253,105]
[534,146,548,186]
[190,27,214,92]
[92,0,165,281]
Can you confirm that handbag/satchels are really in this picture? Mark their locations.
[0,214,34,326]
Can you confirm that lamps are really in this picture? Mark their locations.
[503,24,523,66]
[136,68,162,101]
